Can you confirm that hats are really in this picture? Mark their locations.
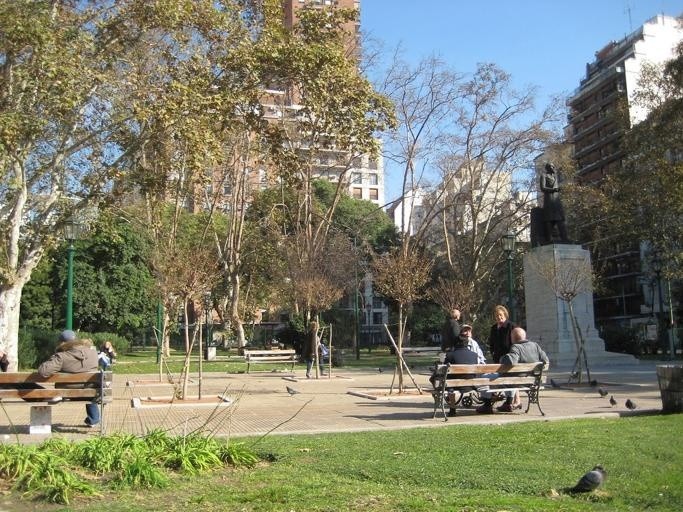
[60,328,76,342]
[460,324,472,333]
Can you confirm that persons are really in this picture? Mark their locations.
[38,329,102,428]
[301,320,328,379]
[539,161,571,242]
[429,303,550,415]
[98,341,116,368]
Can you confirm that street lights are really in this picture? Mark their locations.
[501,226,516,326]
[62,213,80,331]
[366,301,372,353]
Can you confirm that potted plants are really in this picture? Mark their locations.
[656,278,683,414]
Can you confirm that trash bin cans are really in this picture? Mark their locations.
[204,347,216,360]
[656,365,683,412]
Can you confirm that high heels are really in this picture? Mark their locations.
[514,402,522,411]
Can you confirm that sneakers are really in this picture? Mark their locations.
[476,404,493,414]
[85,418,96,428]
[497,402,513,413]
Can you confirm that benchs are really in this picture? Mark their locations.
[0,365,114,435]
[431,361,545,421]
[244,350,298,371]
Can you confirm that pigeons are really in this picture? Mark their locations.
[625,399,637,411]
[572,463,608,492]
[550,378,561,388]
[599,386,608,398]
[379,368,383,372]
[285,386,301,397]
[609,395,618,407]
[590,378,598,388]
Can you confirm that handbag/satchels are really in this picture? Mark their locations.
[320,343,329,357]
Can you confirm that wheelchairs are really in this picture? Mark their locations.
[444,345,484,407]
